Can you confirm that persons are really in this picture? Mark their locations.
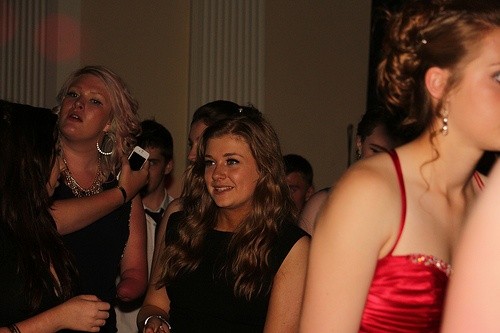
[112,120,176,333]
[281,154,315,221]
[297,101,413,238]
[187,100,242,162]
[42,62,150,333]
[0,99,112,333]
[296,0,499,333]
[136,104,311,333]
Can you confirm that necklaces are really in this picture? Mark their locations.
[56,139,109,198]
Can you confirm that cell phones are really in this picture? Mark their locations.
[116,146,149,180]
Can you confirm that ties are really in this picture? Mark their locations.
[144,208,165,244]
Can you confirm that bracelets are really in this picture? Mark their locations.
[115,184,128,207]
[142,313,172,333]
